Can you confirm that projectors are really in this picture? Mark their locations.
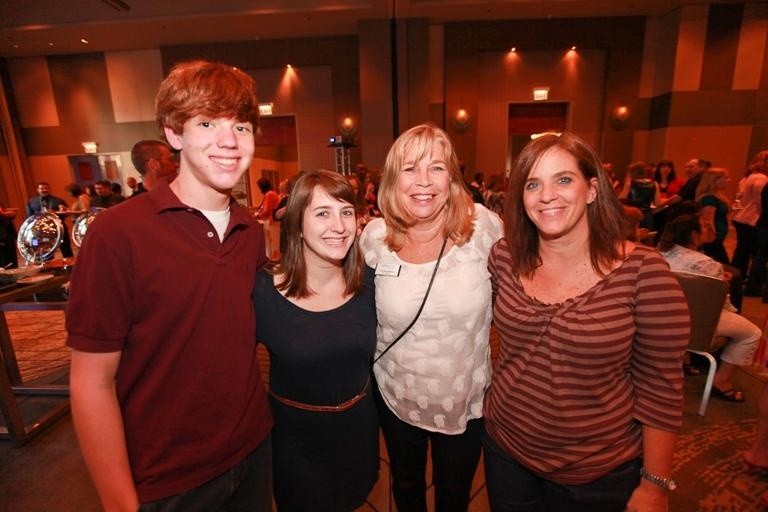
[330,135,345,142]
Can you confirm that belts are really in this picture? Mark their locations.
[270,373,372,412]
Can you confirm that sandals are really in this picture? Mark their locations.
[711,385,746,402]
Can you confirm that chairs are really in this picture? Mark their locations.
[670,269,728,417]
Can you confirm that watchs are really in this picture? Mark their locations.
[638,467,676,493]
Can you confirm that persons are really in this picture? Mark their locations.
[357,122,505,511]
[601,150,767,402]
[468,172,508,215]
[64,61,272,512]
[252,168,295,262]
[481,131,692,512]
[253,169,379,511]
[25,140,180,259]
[347,165,380,219]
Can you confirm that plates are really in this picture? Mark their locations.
[16,274,54,284]
